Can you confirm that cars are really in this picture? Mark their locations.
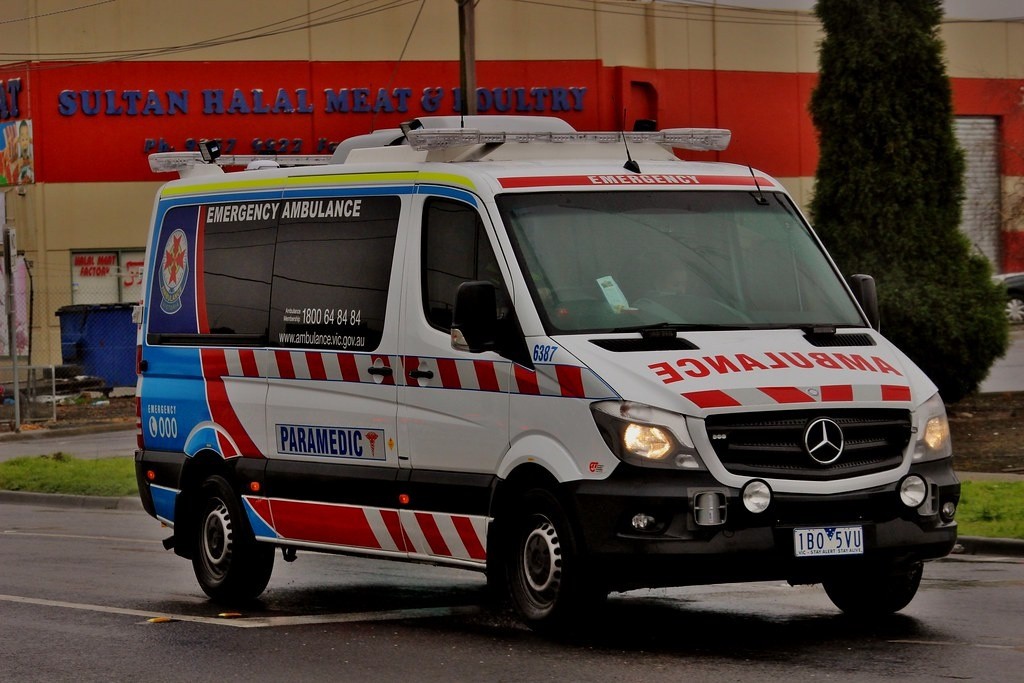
[996,275,1024,325]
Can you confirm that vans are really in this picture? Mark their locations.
[131,113,963,634]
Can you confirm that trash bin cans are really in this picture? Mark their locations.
[100,301,140,388]
[54,303,115,396]
